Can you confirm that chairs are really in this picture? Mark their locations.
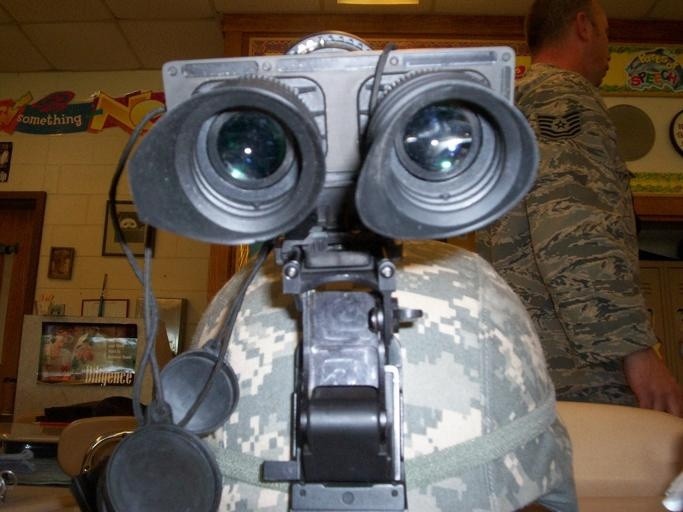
[55,416,137,477]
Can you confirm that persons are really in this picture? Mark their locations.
[474,0,683,418]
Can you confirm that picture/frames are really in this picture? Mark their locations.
[47,247,74,280]
[81,299,129,317]
[102,200,155,257]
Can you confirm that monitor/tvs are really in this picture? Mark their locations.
[36,320,141,388]
